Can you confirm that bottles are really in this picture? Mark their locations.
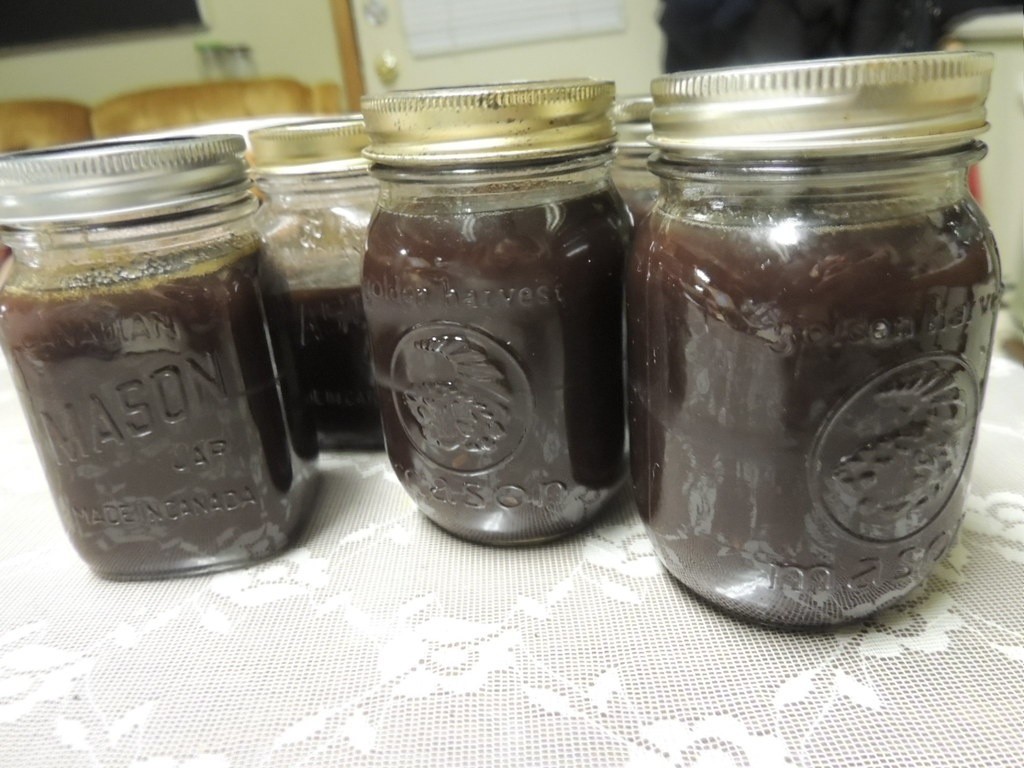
[0,49,1004,632]
